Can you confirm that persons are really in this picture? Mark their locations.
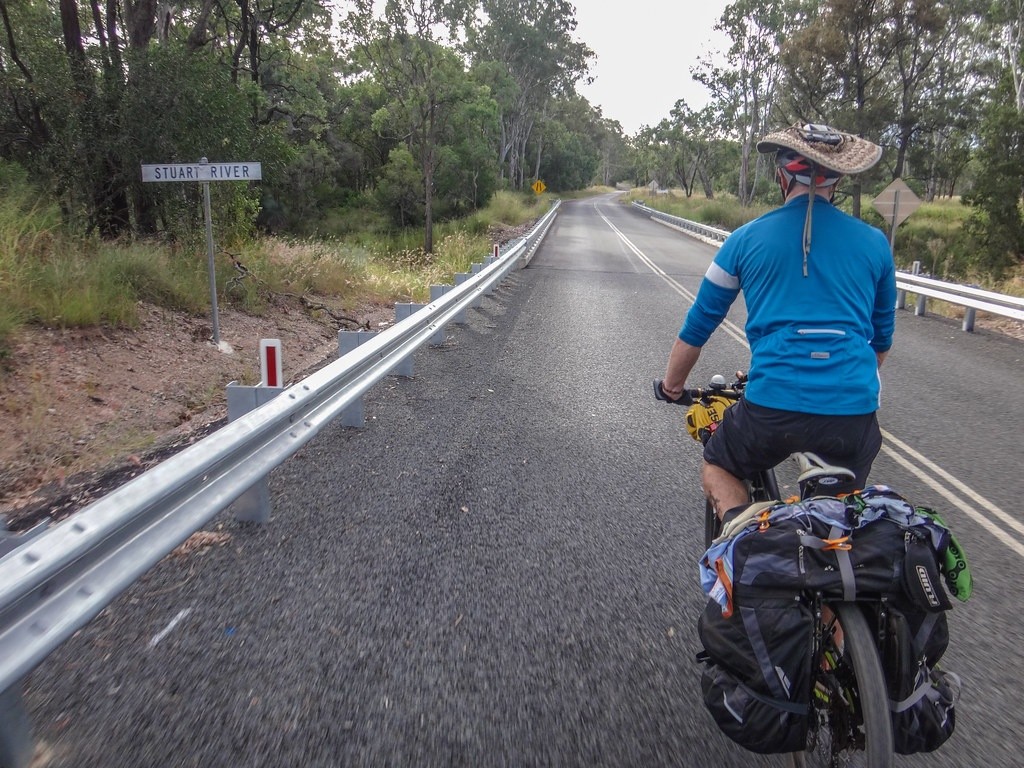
[659,124,899,537]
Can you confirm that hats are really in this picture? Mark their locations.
[756,119,883,187]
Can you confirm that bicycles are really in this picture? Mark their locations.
[653,371,896,768]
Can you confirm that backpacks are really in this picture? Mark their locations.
[696,593,812,753]
[699,487,973,615]
[861,599,956,755]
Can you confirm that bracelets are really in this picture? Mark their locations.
[662,383,684,395]
